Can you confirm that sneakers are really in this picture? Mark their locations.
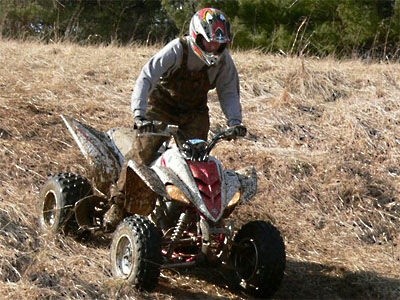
[105,203,125,227]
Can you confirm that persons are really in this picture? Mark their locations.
[103,6,245,231]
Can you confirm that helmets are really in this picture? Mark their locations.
[188,8,231,66]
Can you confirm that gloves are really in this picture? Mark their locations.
[229,125,247,138]
[134,116,154,134]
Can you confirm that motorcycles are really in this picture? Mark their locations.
[36,114,287,299]
